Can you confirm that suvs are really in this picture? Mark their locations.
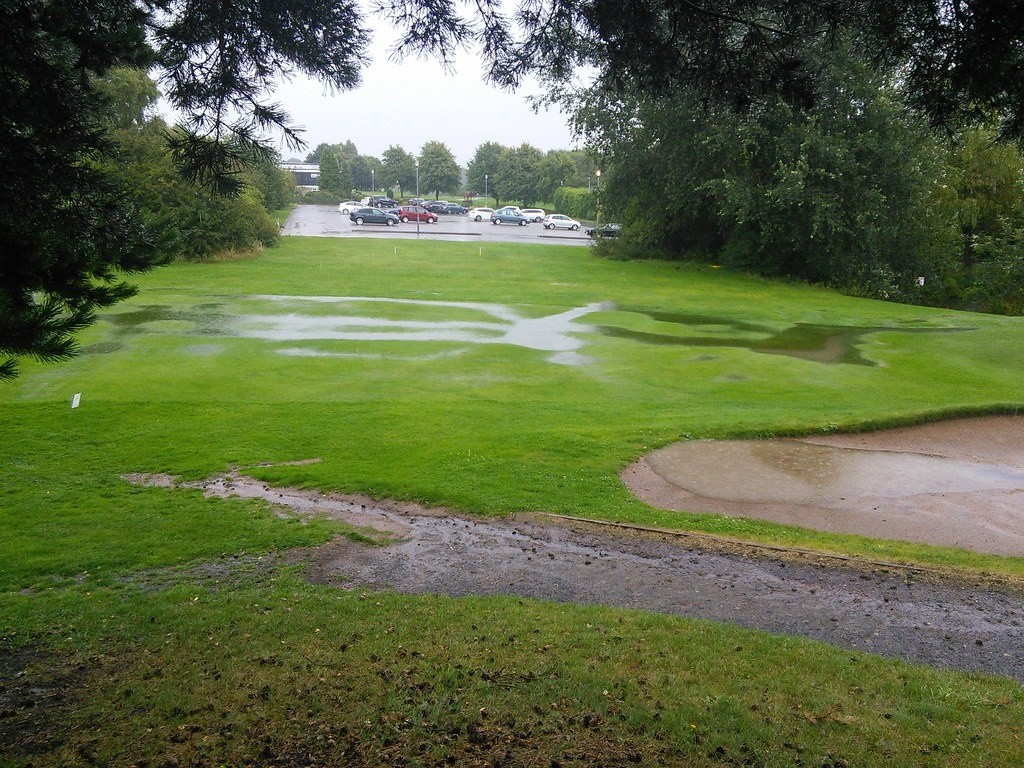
[368,194,399,208]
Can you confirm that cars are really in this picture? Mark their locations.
[467,207,495,222]
[584,222,623,238]
[491,205,531,226]
[339,201,375,215]
[542,214,582,231]
[408,197,468,215]
[400,205,438,224]
[349,207,400,227]
[361,196,371,206]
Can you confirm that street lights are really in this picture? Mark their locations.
[596,170,601,224]
[371,169,375,207]
[414,160,420,239]
[485,175,488,208]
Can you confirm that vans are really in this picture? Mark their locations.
[520,209,546,223]
